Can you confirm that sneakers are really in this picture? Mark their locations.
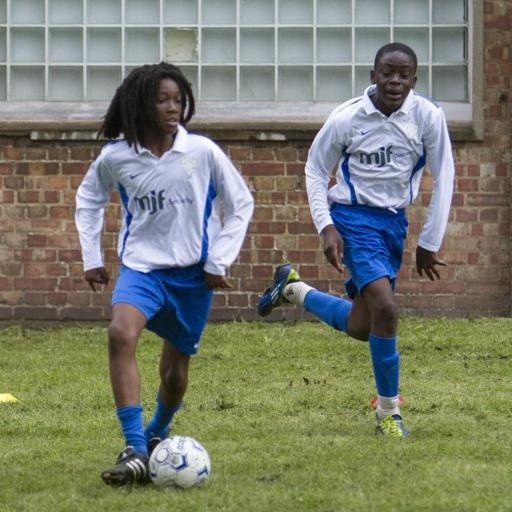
[102,446,153,488]
[375,414,409,441]
[258,263,301,317]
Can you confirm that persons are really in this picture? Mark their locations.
[257,41,458,439]
[72,61,256,487]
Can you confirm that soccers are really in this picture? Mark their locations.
[148,435,211,490]
